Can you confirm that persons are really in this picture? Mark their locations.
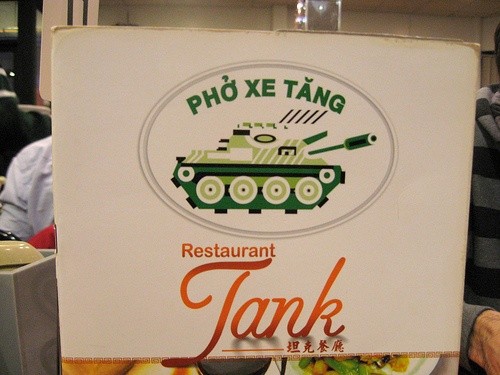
[1,98,53,244]
[460,80,500,375]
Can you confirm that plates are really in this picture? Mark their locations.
[0,241,45,265]
[275,357,441,375]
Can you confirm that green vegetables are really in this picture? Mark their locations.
[297,356,387,375]
